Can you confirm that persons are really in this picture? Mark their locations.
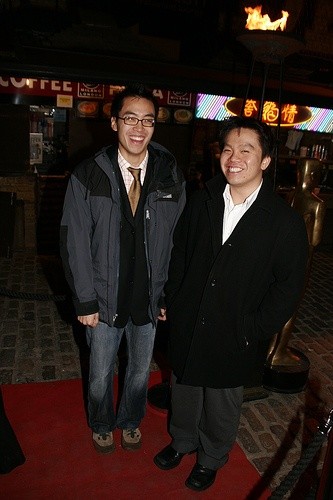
[57,88,186,455]
[264,159,324,365]
[152,117,309,490]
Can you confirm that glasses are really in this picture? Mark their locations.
[116,117,156,127]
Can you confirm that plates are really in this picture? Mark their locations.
[78,101,97,116]
[103,103,112,116]
[157,107,170,121]
[174,109,192,123]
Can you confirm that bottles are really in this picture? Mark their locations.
[312,145,327,158]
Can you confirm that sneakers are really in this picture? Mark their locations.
[121,427,142,450]
[92,432,114,454]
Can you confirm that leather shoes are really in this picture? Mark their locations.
[185,462,218,490]
[152,445,198,470]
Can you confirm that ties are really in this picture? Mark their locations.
[127,167,143,218]
